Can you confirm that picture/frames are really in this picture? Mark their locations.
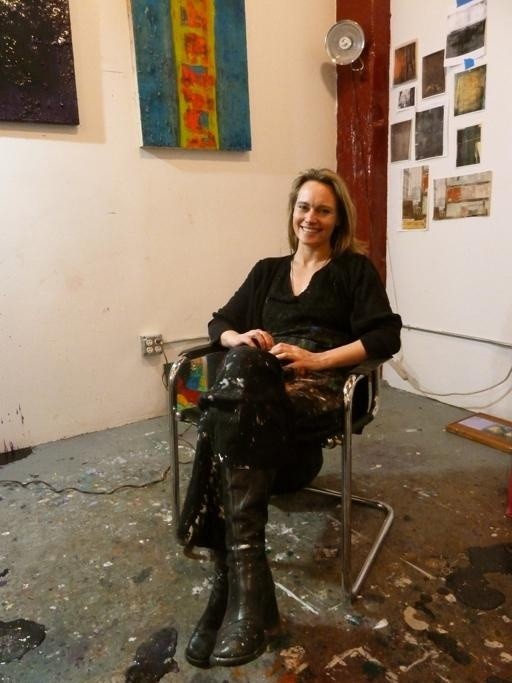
[392,38,419,87]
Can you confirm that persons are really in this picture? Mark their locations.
[171,166,402,669]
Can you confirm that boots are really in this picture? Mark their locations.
[185,463,281,670]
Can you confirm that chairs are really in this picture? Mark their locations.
[163,334,394,603]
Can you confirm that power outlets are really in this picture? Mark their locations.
[142,334,164,357]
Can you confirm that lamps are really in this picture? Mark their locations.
[323,16,367,73]
